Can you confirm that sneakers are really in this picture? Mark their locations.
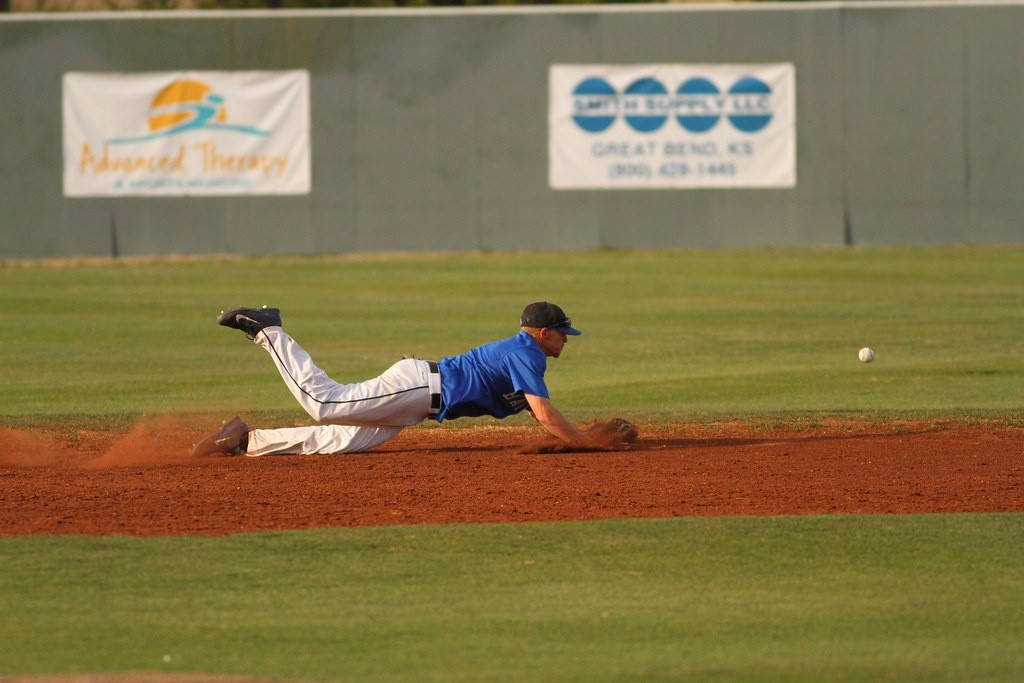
[192,416,251,459]
[216,305,282,338]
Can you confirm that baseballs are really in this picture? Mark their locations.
[859,347,875,363]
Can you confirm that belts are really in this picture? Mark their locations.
[425,360,440,417]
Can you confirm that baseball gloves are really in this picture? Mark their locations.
[600,416,640,443]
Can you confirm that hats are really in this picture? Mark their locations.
[519,301,582,337]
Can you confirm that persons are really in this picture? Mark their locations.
[190,302,638,457]
